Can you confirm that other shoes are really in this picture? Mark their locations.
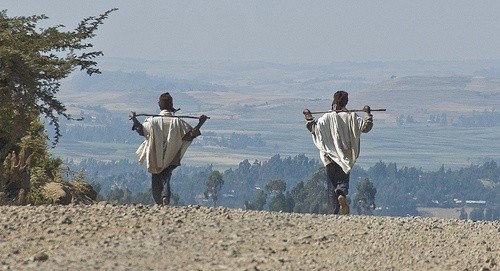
[338,194,349,214]
[163,197,168,206]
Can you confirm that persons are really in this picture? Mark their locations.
[303,91,373,217]
[129,93,208,205]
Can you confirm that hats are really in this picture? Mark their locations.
[158,92,173,109]
[332,91,348,107]
